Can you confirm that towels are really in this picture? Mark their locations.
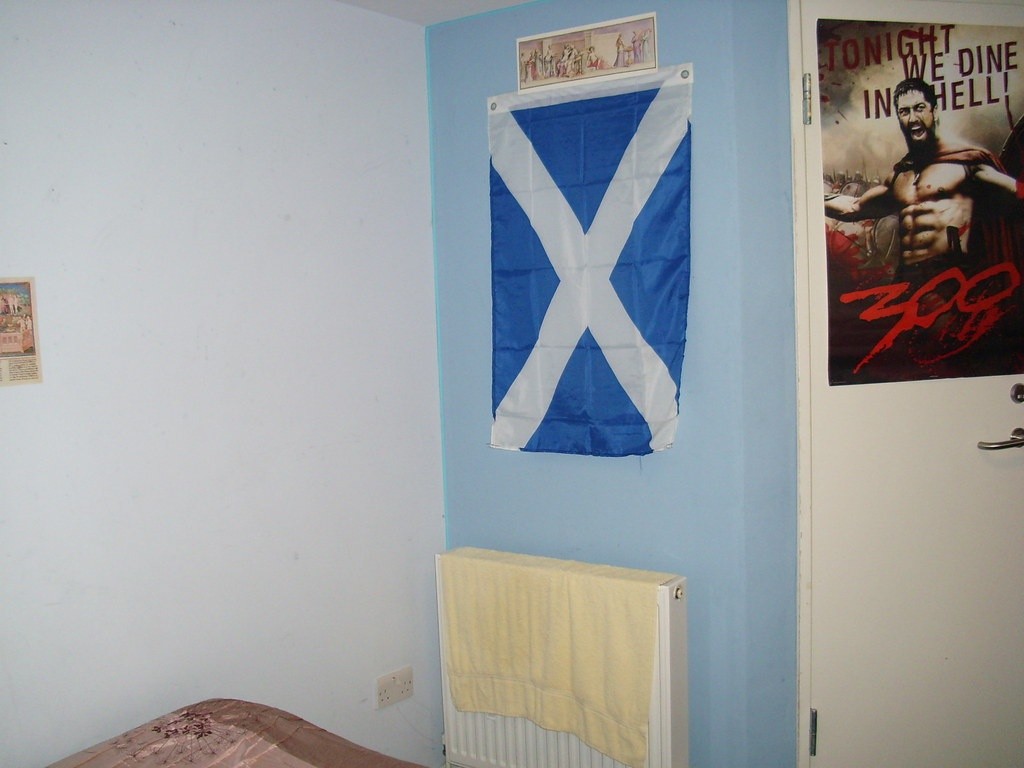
[441,546,678,768]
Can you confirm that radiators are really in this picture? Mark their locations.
[434,547,688,767]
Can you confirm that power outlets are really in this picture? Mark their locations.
[376,666,414,710]
